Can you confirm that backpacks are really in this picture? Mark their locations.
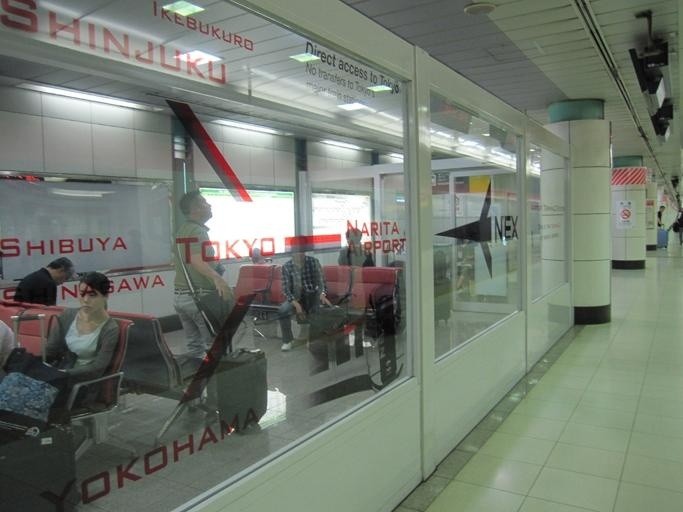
[0,346,68,439]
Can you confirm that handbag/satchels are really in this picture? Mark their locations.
[192,285,242,337]
[46,315,78,371]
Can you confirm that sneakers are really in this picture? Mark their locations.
[280,339,294,351]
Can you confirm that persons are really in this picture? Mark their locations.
[15,257,73,306]
[277,239,333,352]
[42,272,119,424]
[455,235,476,296]
[172,190,235,418]
[656,205,683,246]
[337,228,375,267]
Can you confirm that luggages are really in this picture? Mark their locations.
[308,304,352,369]
[657,224,669,249]
[206,347,268,433]
